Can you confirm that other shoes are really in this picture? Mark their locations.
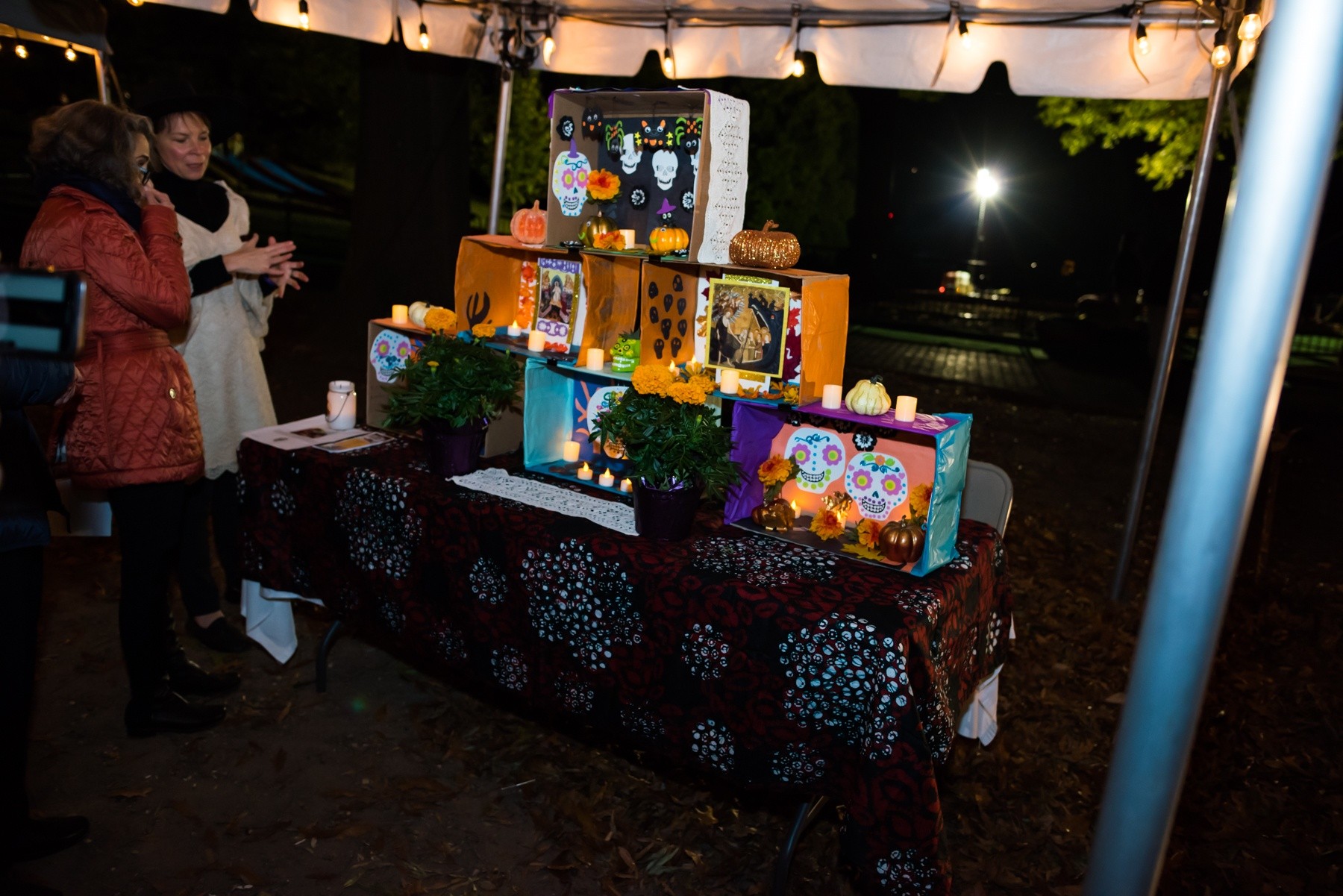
[185,582,263,652]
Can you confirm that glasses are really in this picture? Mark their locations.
[134,163,153,186]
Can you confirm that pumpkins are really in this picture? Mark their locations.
[874,513,925,566]
[508,197,553,246]
[650,222,690,256]
[726,220,805,270]
[578,211,619,251]
[843,373,892,420]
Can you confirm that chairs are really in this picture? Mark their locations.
[962,458,1011,772]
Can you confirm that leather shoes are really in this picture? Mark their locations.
[123,690,230,737]
[171,659,239,698]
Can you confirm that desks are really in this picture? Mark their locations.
[233,414,1018,896]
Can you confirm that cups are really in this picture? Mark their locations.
[327,380,357,430]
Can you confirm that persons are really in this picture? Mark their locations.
[112,110,312,661]
[19,102,245,745]
